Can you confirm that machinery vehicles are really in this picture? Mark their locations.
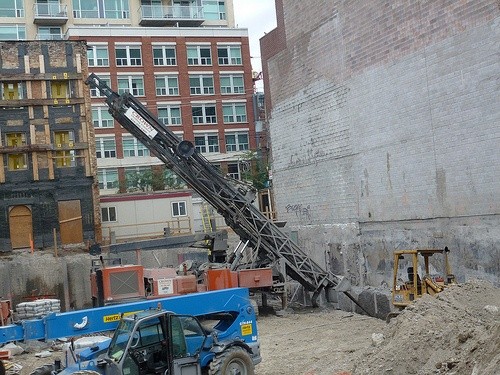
[386,246,456,325]
[0,286,261,375]
